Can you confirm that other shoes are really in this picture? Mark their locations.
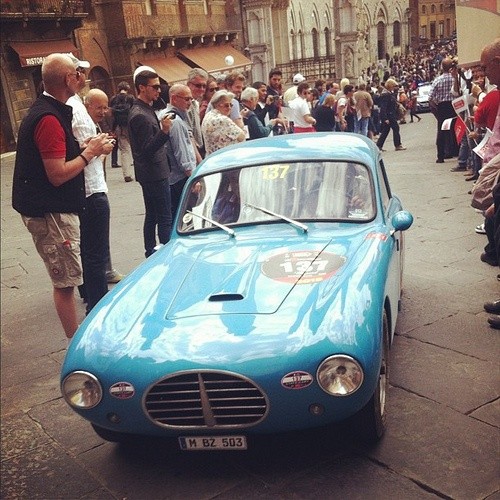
[104,269,125,283]
[484,298,500,314]
[464,174,479,181]
[480,252,499,266]
[487,316,500,327]
[380,148,387,152]
[436,159,444,163]
[475,224,486,234]
[450,164,466,171]
[125,177,133,182]
[395,145,408,151]
[112,164,121,168]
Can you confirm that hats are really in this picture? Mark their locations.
[293,73,306,83]
[63,54,90,70]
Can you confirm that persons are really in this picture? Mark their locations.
[383,30,457,93]
[126,66,287,258]
[108,80,139,182]
[212,169,264,226]
[10,52,110,345]
[283,73,421,152]
[102,107,121,168]
[299,165,372,220]
[427,38,500,328]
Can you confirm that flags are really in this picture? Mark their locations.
[454,117,466,143]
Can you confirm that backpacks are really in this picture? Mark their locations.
[332,95,344,116]
[112,94,131,136]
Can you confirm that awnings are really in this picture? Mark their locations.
[139,56,192,86]
[180,45,253,80]
[11,40,81,67]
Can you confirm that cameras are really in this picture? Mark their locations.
[271,96,281,100]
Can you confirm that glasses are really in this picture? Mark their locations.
[80,68,86,73]
[176,95,193,101]
[218,103,234,108]
[146,84,161,90]
[190,82,207,88]
[208,86,220,92]
[303,91,310,95]
[70,72,81,79]
[480,61,491,72]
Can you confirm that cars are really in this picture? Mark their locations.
[416,85,431,113]
[60,132,414,449]
